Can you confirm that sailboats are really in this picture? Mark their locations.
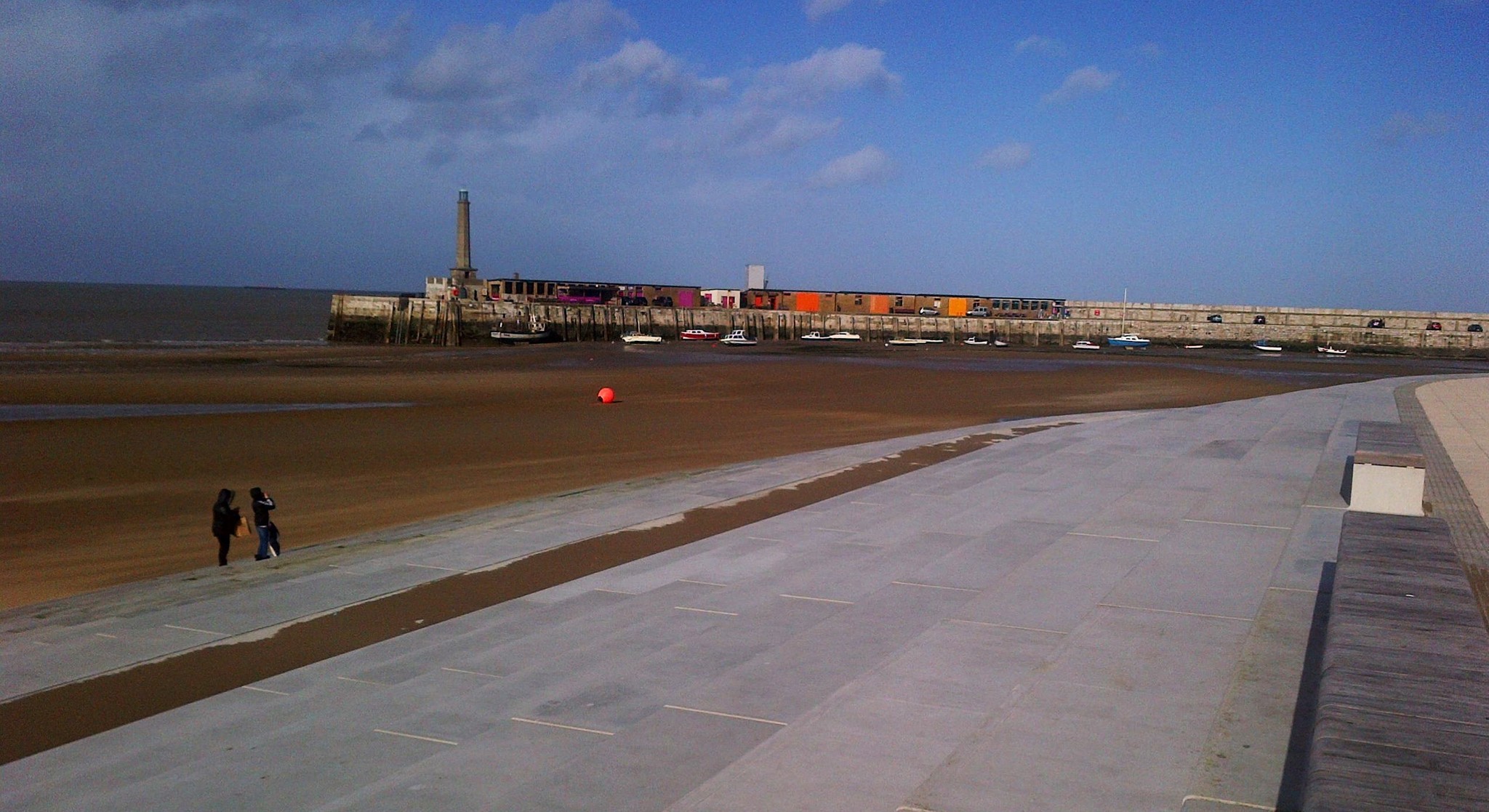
[1108,287,1151,347]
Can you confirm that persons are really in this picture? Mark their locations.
[212,488,242,566]
[250,486,275,560]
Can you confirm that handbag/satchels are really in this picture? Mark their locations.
[232,514,252,537]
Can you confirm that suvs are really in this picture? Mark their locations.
[1254,316,1266,324]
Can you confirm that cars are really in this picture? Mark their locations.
[919,307,940,316]
[1468,324,1483,331]
[966,307,987,316]
[1426,322,1442,330]
[1210,315,1222,323]
[1368,319,1383,328]
[622,296,673,306]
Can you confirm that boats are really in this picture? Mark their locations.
[491,310,552,344]
[801,331,832,340]
[681,330,719,341]
[1185,344,1204,348]
[995,340,1009,347]
[1252,344,1282,351]
[622,318,662,343]
[1072,340,1100,349]
[888,338,944,344]
[963,336,988,344]
[1323,347,1348,354]
[829,331,861,341]
[719,329,757,346]
[1317,341,1329,351]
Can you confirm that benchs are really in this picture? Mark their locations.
[1350,421,1426,517]
